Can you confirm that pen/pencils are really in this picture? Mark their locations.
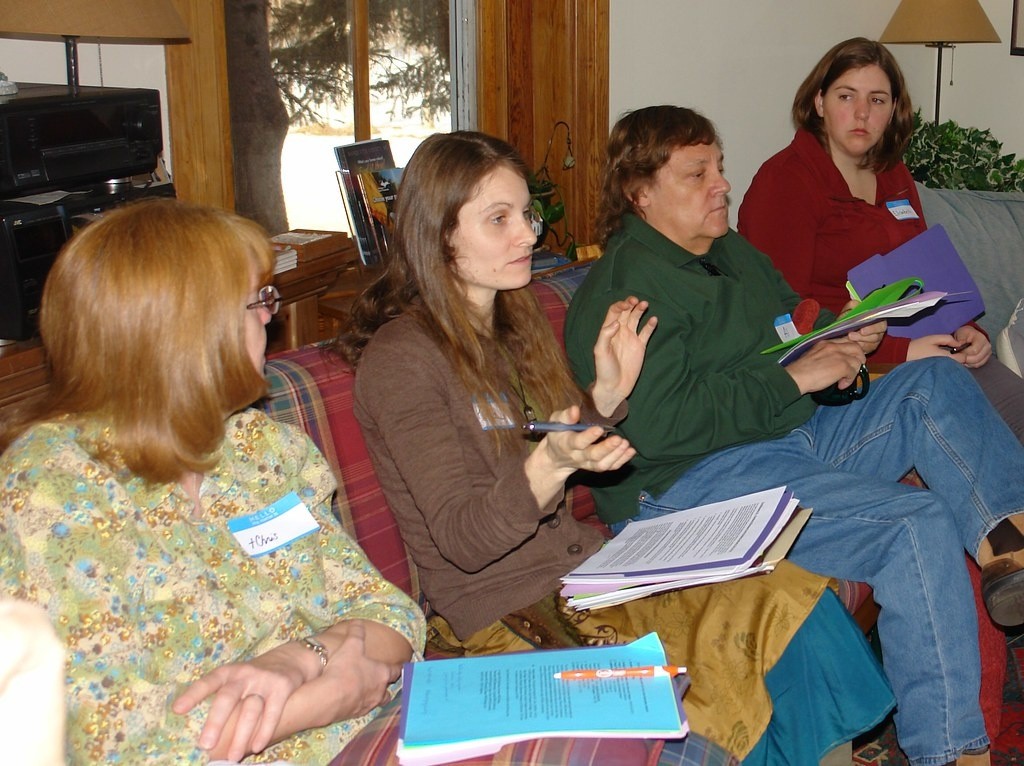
[950,343,971,354]
[553,667,689,679]
[520,421,616,433]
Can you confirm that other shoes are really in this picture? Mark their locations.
[981,557,1024,626]
[944,745,990,766]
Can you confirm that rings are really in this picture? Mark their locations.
[243,694,266,707]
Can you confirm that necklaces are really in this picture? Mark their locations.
[182,473,197,496]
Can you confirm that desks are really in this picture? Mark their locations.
[0,250,351,457]
[317,248,596,320]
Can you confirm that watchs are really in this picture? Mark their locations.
[287,637,328,665]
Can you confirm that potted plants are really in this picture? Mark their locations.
[528,171,586,262]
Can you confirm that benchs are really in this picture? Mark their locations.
[253,253,882,634]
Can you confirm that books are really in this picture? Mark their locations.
[396,630,692,766]
[567,481,815,612]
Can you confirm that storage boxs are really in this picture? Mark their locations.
[269,229,350,263]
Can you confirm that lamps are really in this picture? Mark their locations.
[0,0,191,86]
[876,0,1002,127]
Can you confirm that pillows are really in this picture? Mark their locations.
[325,645,692,766]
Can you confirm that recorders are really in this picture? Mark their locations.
[0,81,163,200]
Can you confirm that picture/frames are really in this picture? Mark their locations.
[1010,0,1024,56]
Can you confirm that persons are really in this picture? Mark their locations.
[565,109,1024,766]
[358,131,896,766]
[0,200,735,766]
[737,37,1024,450]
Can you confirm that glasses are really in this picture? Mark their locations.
[246,285,281,315]
